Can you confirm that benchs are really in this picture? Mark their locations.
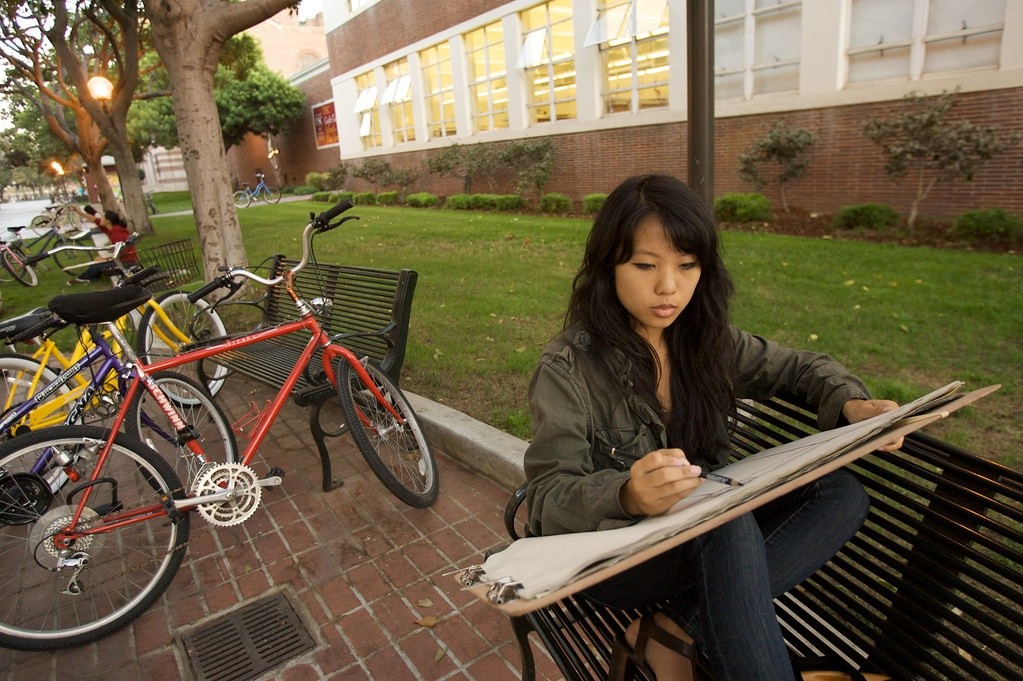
[191,254,418,493]
[484,389,1023,681]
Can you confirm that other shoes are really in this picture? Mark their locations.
[66,276,89,285]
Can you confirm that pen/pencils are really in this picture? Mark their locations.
[611,446,745,487]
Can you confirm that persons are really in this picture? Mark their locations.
[66,205,137,286]
[49,184,87,204]
[522,172,905,681]
[138,169,157,215]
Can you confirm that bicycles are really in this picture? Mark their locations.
[0,199,94,287]
[233,173,281,208]
[0,229,229,503]
[0,198,440,652]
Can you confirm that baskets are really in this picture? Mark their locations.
[120,239,200,294]
